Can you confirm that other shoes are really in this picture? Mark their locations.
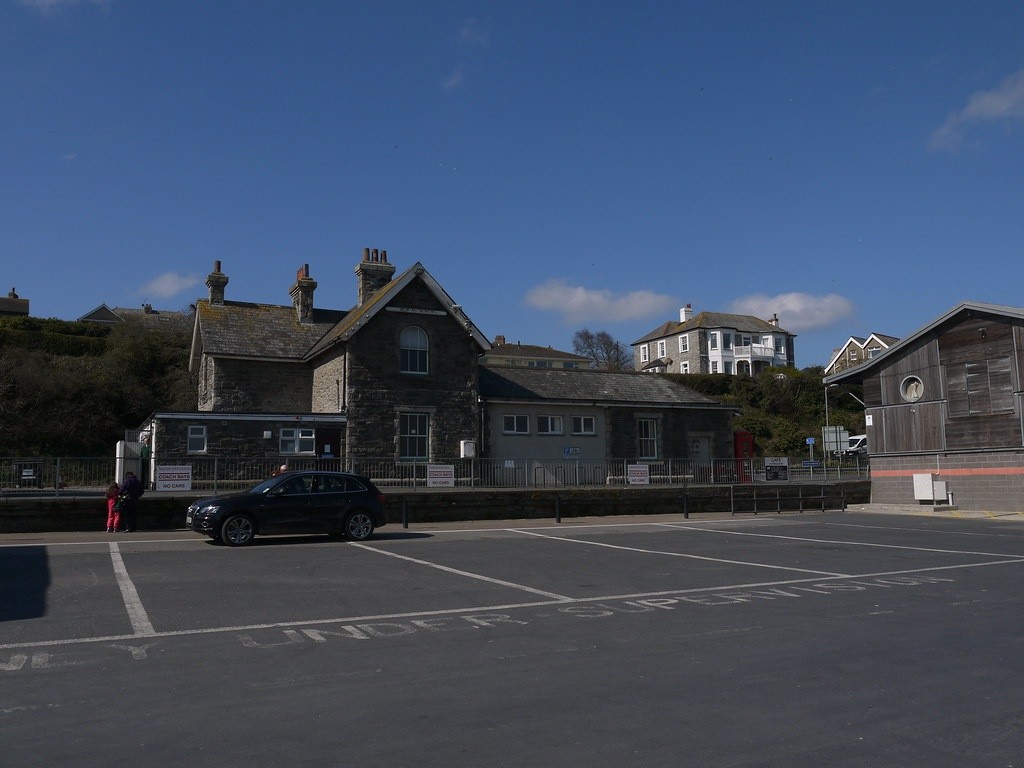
[105,529,111,532]
[114,529,119,532]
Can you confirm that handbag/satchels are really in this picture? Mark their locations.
[114,497,122,513]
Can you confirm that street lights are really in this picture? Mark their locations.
[824,383,840,463]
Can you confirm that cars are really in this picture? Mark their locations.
[21,469,37,486]
[185,469,390,547]
[832,435,868,460]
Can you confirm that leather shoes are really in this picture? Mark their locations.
[123,530,132,532]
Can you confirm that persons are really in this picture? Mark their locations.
[119,472,145,533]
[294,480,303,493]
[141,438,151,488]
[271,465,288,477]
[105,481,121,533]
